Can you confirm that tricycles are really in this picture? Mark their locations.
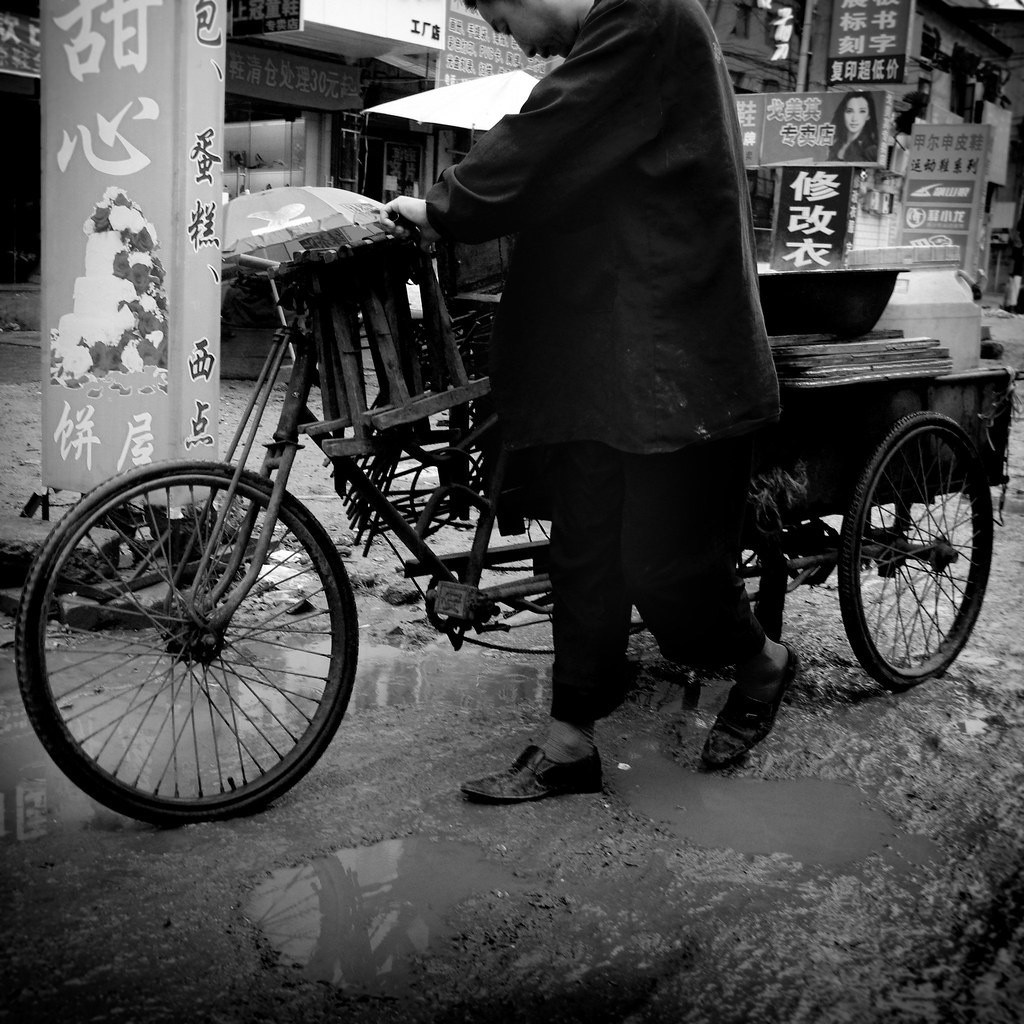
[10,194,1024,829]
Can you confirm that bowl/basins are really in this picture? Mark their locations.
[757,269,911,339]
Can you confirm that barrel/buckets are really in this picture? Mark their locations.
[848,244,982,373]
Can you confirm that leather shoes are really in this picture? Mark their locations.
[703,639,801,767]
[461,743,603,801]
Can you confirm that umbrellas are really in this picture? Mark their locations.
[217,184,393,368]
[359,69,541,134]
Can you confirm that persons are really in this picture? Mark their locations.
[823,91,878,163]
[381,0,798,804]
